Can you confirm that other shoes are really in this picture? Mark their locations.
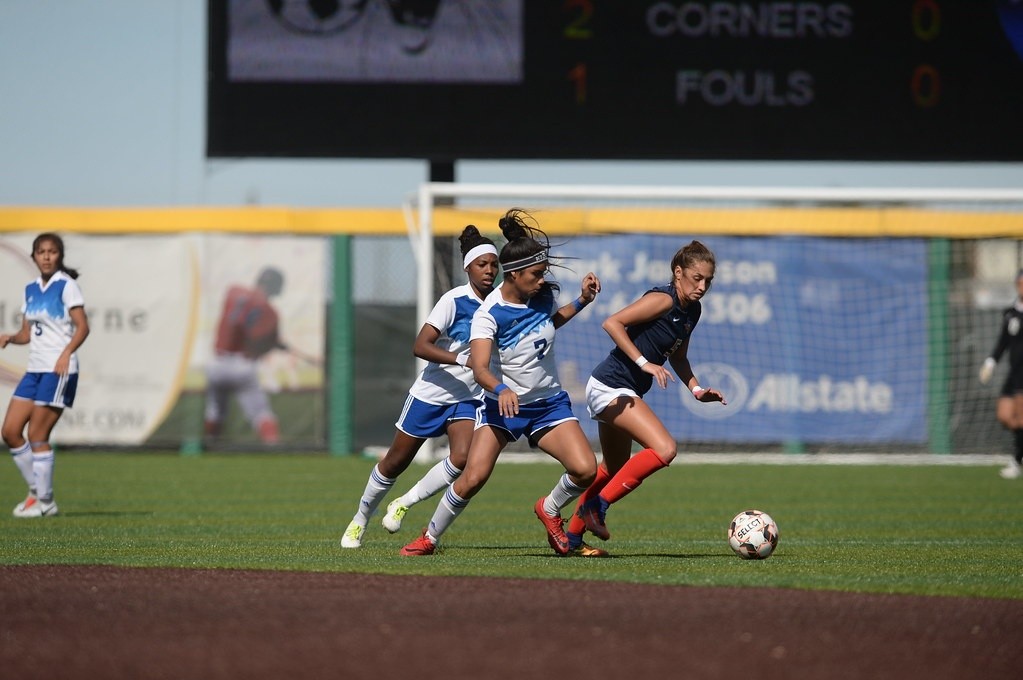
[998,463,1023,479]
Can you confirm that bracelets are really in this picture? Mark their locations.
[571,298,585,313]
[692,385,702,395]
[494,383,510,396]
[455,353,470,366]
[635,355,648,368]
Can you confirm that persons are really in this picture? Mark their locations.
[340,225,499,548]
[565,239,727,557]
[399,206,602,556]
[978,267,1023,479]
[0,232,89,518]
[200,266,283,455]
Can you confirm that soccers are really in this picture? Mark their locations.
[728,510,779,559]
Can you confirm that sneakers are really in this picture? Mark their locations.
[575,501,610,540]
[400,528,436,557]
[567,541,607,558]
[340,518,366,548]
[534,497,569,556]
[382,498,408,533]
[13,494,37,516]
[22,497,57,518]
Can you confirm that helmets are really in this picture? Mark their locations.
[257,269,282,296]
